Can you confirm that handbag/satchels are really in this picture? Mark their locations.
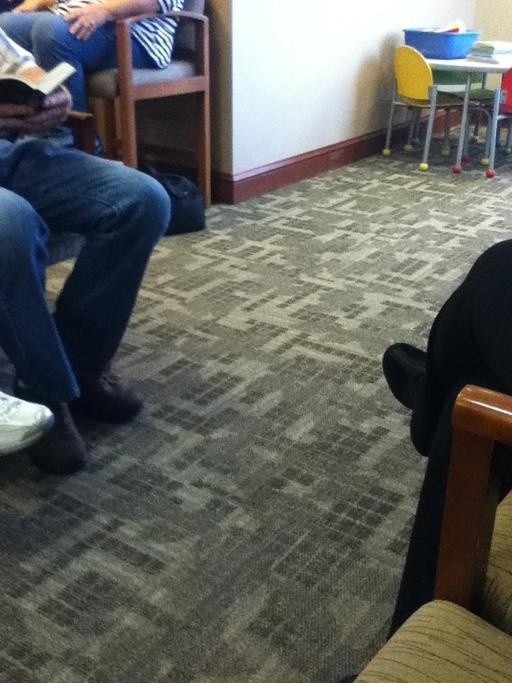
[147,162,203,234]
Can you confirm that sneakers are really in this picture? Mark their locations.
[78,373,146,424]
[384,343,427,407]
[1,380,89,476]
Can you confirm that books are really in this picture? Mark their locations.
[0,58,77,144]
[469,37,511,65]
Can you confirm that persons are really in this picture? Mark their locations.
[335,230,509,683]
[0,22,173,480]
[0,0,189,178]
[0,386,58,458]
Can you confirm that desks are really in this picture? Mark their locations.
[425,58,512,179]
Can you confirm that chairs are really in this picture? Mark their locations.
[382,45,472,170]
[351,384,512,683]
[440,66,512,165]
[86,0,214,210]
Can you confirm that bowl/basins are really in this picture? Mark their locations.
[403,27,478,60]
